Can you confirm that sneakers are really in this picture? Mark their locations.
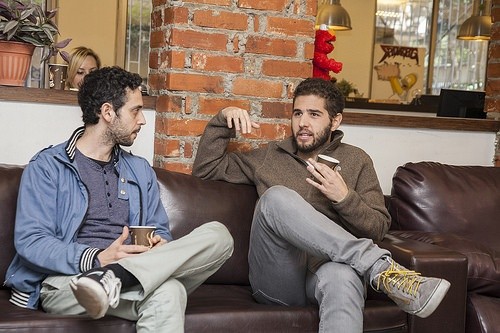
[69,267,122,319]
[369,255,452,319]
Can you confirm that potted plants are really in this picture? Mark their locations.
[0,0,71,88]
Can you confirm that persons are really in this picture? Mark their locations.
[62,46,101,91]
[3,66,234,333]
[193,77,452,333]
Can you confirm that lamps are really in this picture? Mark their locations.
[455,0,493,43]
[315,0,353,32]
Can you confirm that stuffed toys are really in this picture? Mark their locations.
[312,23,342,81]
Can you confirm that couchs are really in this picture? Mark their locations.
[0,161,469,333]
[381,162,500,333]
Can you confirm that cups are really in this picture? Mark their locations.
[314,154,340,185]
[129,225,158,254]
[48,64,68,91]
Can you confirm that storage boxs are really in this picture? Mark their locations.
[435,89,486,119]
